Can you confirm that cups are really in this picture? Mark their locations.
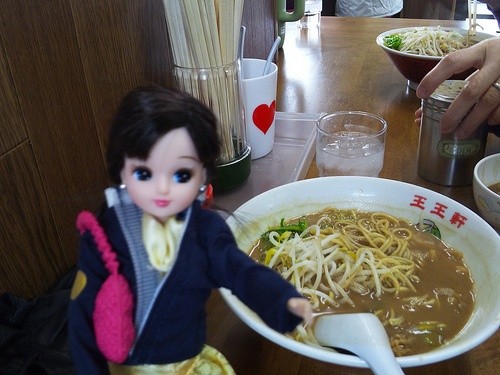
[277,0,305,48]
[298,0,322,28]
[241,59,278,159]
[172,58,252,193]
[317,110,386,178]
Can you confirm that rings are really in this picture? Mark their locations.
[493,83,500,90]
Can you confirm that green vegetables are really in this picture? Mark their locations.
[262,215,307,242]
[382,34,402,51]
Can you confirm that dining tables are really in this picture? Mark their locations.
[205,16,500,375]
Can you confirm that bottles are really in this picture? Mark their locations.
[416,80,489,186]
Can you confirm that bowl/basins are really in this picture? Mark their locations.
[473,153,500,230]
[376,27,496,91]
[218,176,500,368]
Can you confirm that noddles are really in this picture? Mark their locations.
[248,207,475,356]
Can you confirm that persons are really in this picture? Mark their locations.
[68,84,312,375]
[414,0,500,139]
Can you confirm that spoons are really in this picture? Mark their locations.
[315,313,404,375]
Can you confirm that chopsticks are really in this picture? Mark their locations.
[163,0,245,164]
[467,0,477,36]
